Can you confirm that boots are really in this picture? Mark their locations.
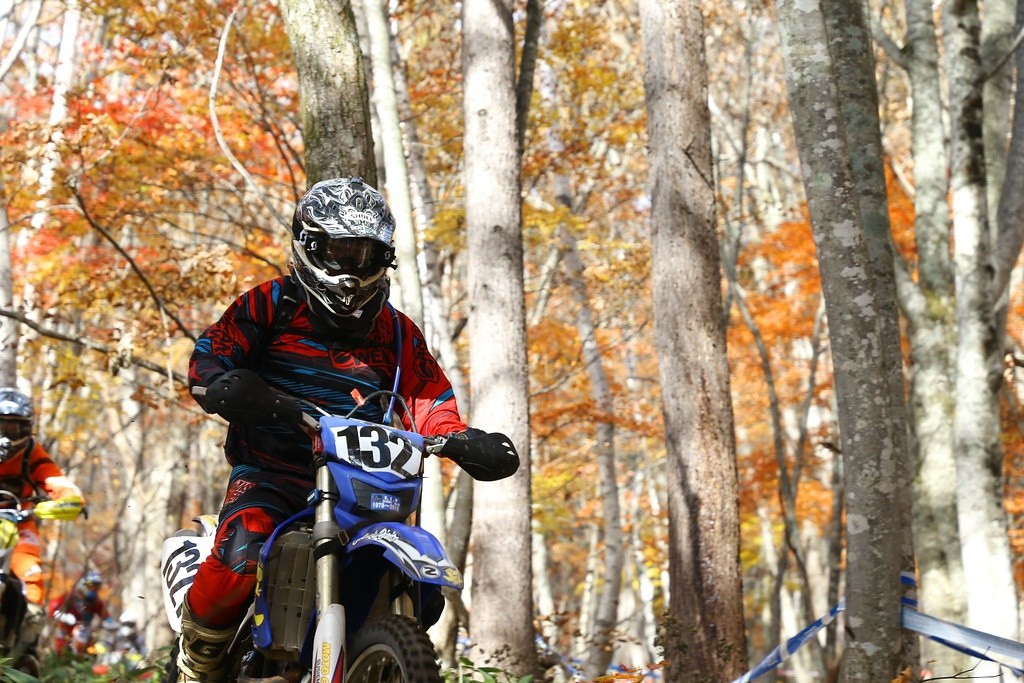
[171,587,249,683]
[6,603,46,679]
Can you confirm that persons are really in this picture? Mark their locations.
[1,386,85,667]
[49,571,112,662]
[177,177,489,683]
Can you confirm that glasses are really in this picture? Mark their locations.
[290,211,395,280]
[0,416,32,445]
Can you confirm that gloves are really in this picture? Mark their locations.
[455,427,488,440]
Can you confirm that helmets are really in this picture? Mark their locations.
[0,387,35,462]
[77,570,102,599]
[291,175,398,317]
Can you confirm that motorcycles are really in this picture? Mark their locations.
[0,489,157,683]
[157,386,519,683]
